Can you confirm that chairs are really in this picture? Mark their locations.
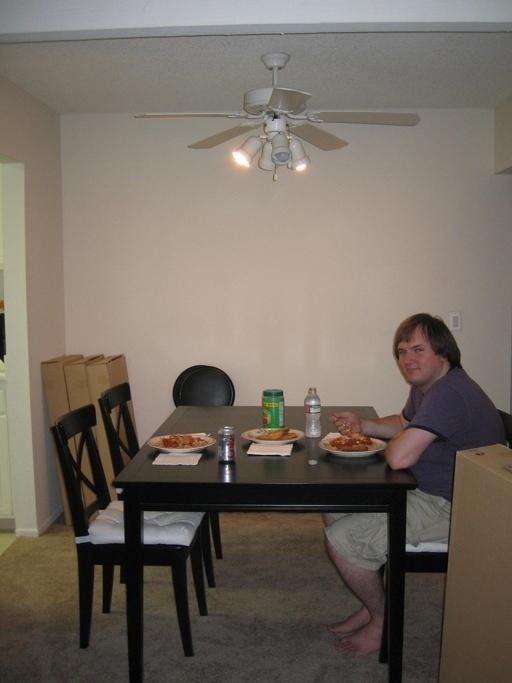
[378,409,512,661]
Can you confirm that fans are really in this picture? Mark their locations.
[133,50,422,151]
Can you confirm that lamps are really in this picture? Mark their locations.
[231,119,310,181]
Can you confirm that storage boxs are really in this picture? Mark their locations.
[439,443,511,682]
[40,354,140,526]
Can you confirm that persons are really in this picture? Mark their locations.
[322,308,506,659]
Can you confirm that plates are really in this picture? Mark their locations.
[145,432,217,454]
[317,431,388,458]
[240,426,306,446]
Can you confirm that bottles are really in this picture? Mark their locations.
[304,386,323,440]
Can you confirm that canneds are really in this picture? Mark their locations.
[217,425,236,463]
